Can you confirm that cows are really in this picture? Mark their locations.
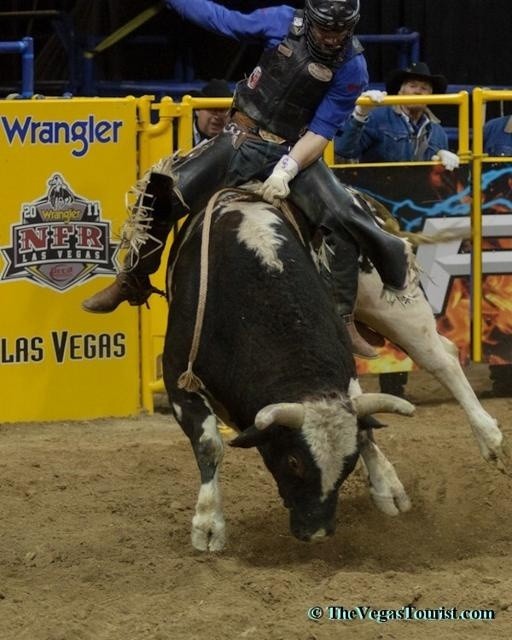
[162,187,512,551]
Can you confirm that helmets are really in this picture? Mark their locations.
[302,0,360,65]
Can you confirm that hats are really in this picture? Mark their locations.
[385,61,447,94]
[187,78,232,96]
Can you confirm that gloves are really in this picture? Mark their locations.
[257,154,299,209]
[431,149,459,172]
[355,89,387,116]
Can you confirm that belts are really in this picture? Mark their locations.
[230,109,288,146]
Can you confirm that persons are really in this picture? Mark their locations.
[472,117,512,398]
[189,82,235,146]
[84,0,378,359]
[331,60,462,406]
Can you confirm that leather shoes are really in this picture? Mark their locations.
[338,313,379,360]
[83,274,152,314]
